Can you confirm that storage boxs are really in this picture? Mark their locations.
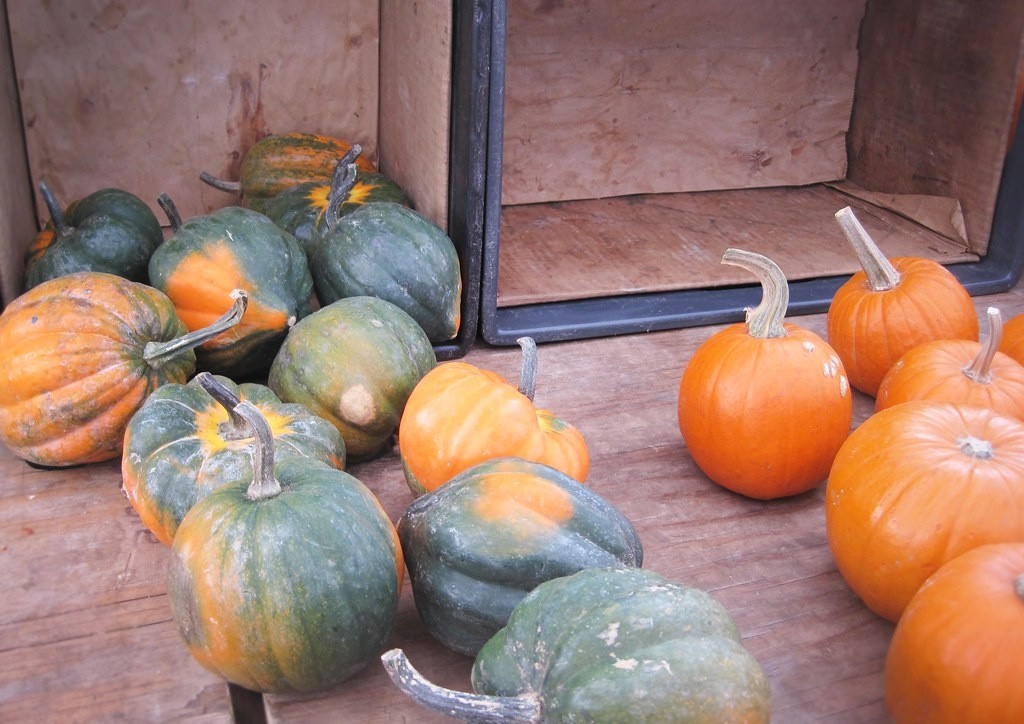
[481,2,1019,351]
[0,1,493,360]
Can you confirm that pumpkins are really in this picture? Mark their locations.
[381,567,772,724]
[874,307,1024,424]
[396,458,644,657]
[120,371,345,546]
[677,247,852,500]
[165,403,404,694]
[399,337,589,499]
[0,132,462,467]
[882,541,1024,724]
[825,400,1024,624]
[826,205,979,397]
[994,312,1024,367]
